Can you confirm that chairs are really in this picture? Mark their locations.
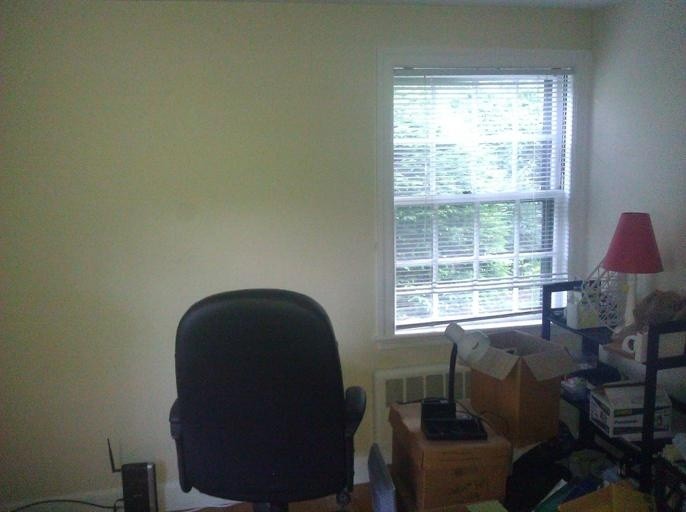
[170,288,365,511]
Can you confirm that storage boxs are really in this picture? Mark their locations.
[466,327,578,447]
[390,400,513,511]
[557,480,652,510]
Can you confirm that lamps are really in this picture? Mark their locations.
[602,211,662,355]
[420,323,494,441]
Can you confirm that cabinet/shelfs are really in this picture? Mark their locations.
[542,279,686,501]
